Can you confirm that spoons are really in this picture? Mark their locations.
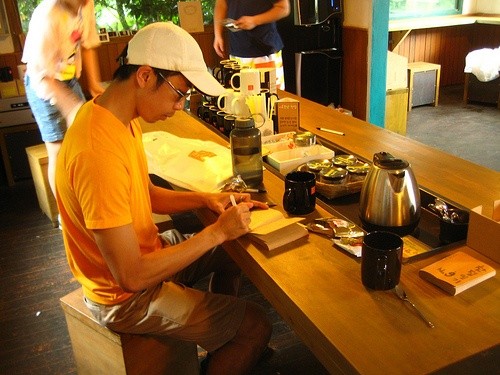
[394,285,435,328]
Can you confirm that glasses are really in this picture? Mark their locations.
[154,66,190,102]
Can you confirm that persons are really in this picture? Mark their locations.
[21,0,103,230]
[55,21,272,375]
[213,0,290,92]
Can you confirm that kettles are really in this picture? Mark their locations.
[358,152,421,235]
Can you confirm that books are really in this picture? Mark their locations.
[418,251,496,297]
[242,208,309,251]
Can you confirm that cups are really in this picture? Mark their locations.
[361,231,403,290]
[283,171,316,214]
[230,113,265,189]
[197,60,261,137]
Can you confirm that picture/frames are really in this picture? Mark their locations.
[99,27,138,42]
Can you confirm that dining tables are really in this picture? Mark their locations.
[138,89,500,375]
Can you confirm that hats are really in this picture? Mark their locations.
[120,20,228,96]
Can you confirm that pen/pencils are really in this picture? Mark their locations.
[245,189,266,193]
[230,195,236,206]
[317,128,345,136]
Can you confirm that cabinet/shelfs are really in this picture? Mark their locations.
[0,122,44,189]
[0,0,24,54]
[385,88,409,135]
[77,23,230,101]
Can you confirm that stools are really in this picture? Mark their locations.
[409,62,442,111]
[58,285,199,375]
[462,71,500,108]
[25,143,59,228]
